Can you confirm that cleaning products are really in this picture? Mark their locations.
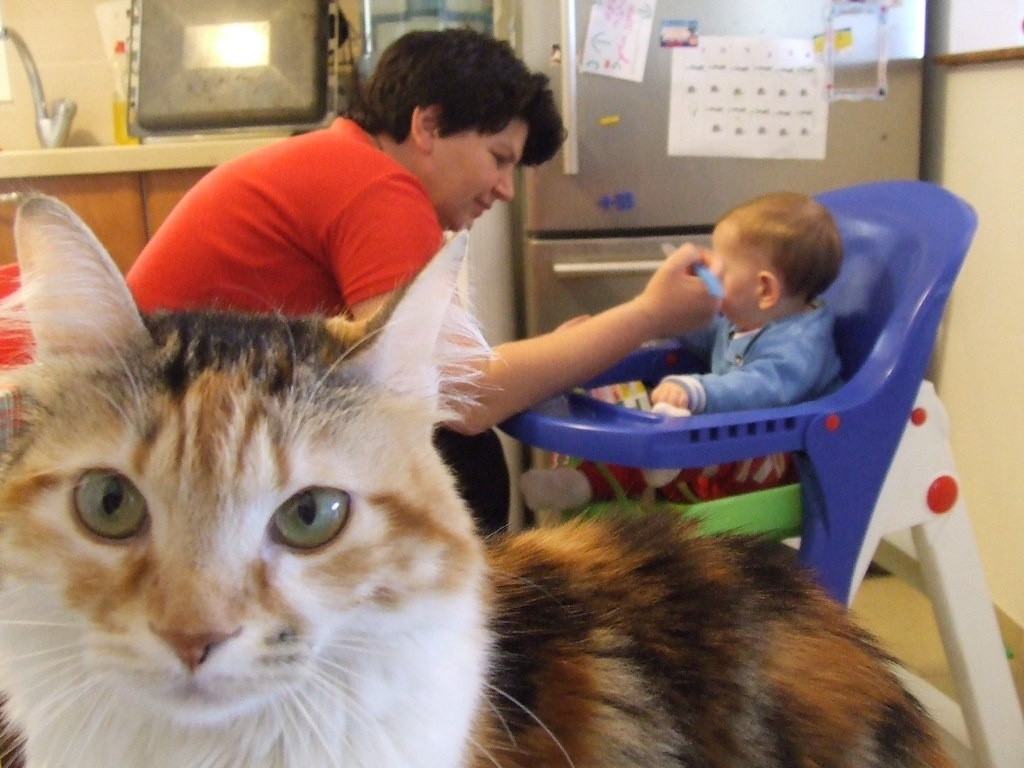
[110,38,134,147]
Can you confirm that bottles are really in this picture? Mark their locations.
[358,0,494,112]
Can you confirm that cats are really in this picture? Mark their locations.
[0,194,961,768]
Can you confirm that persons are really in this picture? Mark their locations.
[125,27,727,533]
[518,192,843,510]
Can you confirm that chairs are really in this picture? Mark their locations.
[491,180,1024,767]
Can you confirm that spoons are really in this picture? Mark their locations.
[661,244,724,298]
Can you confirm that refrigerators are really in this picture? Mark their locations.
[489,0,925,345]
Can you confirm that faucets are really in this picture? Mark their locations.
[1,26,77,148]
